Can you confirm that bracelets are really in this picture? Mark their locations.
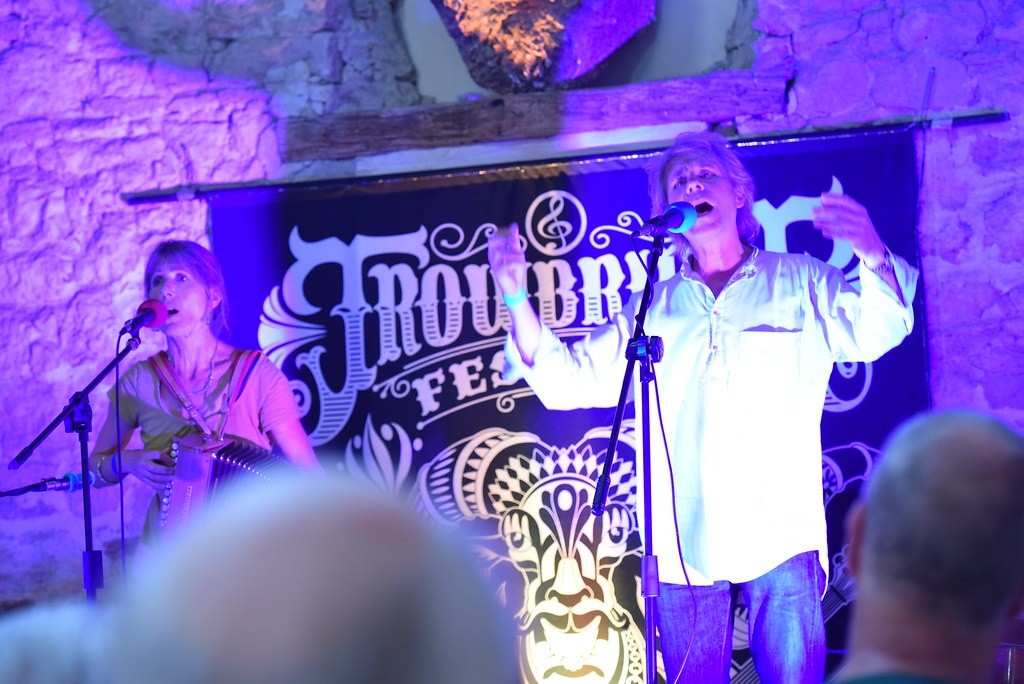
[503,288,527,307]
[111,450,127,478]
[95,455,116,486]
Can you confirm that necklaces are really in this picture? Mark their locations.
[165,340,217,395]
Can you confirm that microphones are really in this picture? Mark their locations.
[118,299,167,335]
[31,471,95,495]
[632,201,697,238]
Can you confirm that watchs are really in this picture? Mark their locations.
[867,246,894,273]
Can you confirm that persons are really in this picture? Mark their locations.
[102,464,521,684]
[832,402,1024,684]
[488,127,919,684]
[88,239,321,545]
